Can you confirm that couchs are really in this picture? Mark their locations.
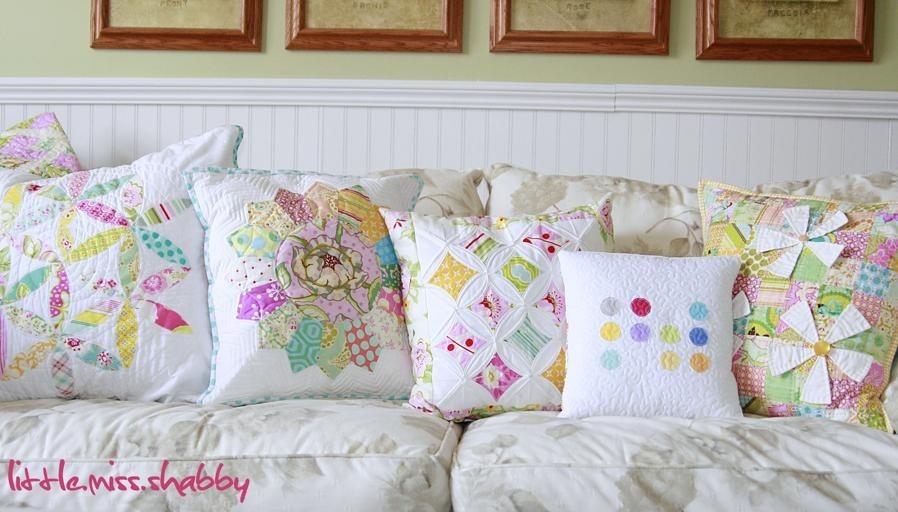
[0,376,897,509]
